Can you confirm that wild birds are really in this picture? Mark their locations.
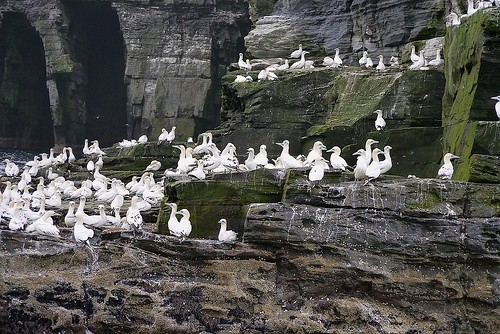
[0,0,500,245]
